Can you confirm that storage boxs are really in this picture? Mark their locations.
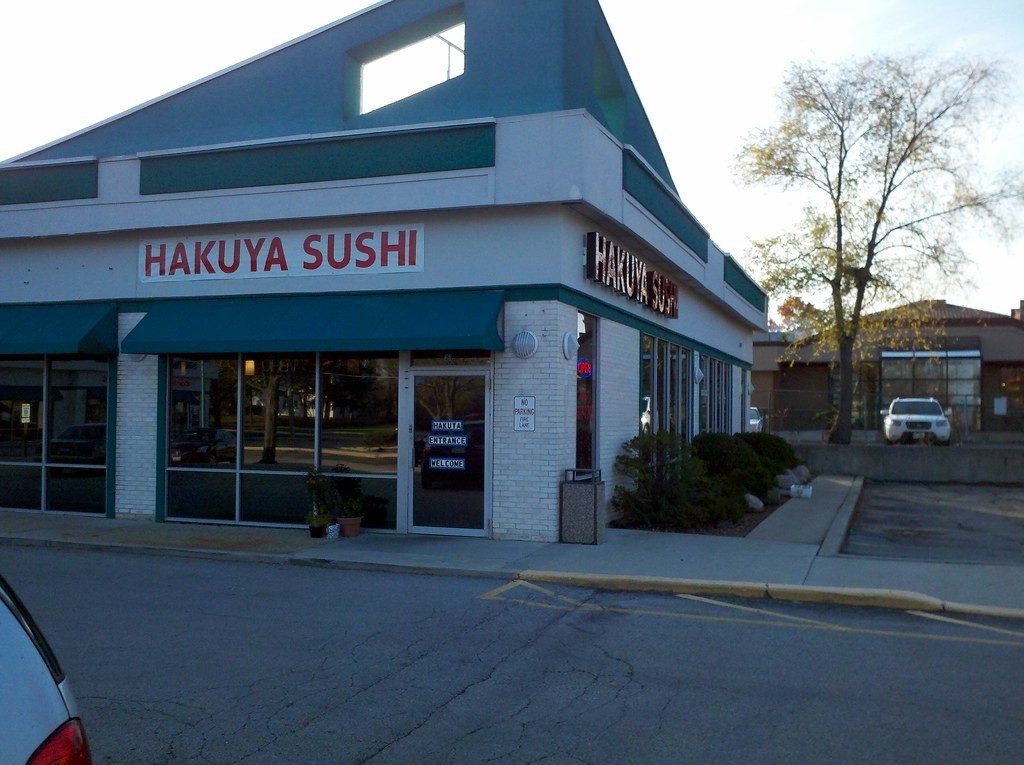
[790,485,813,499]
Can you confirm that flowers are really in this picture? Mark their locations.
[305,467,332,502]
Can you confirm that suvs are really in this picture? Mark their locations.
[641,395,652,436]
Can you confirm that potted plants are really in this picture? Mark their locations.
[337,496,363,537]
[304,512,326,539]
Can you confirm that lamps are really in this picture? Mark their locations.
[510,330,538,360]
[748,383,755,395]
[562,332,581,361]
[695,368,705,384]
[245,360,255,376]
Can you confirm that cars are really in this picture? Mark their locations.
[749,406,765,434]
[0,576,95,765]
[168,428,236,467]
[881,396,953,447]
[40,423,107,478]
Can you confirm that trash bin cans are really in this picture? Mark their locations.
[559,468,607,546]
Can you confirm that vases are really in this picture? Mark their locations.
[320,516,335,535]
